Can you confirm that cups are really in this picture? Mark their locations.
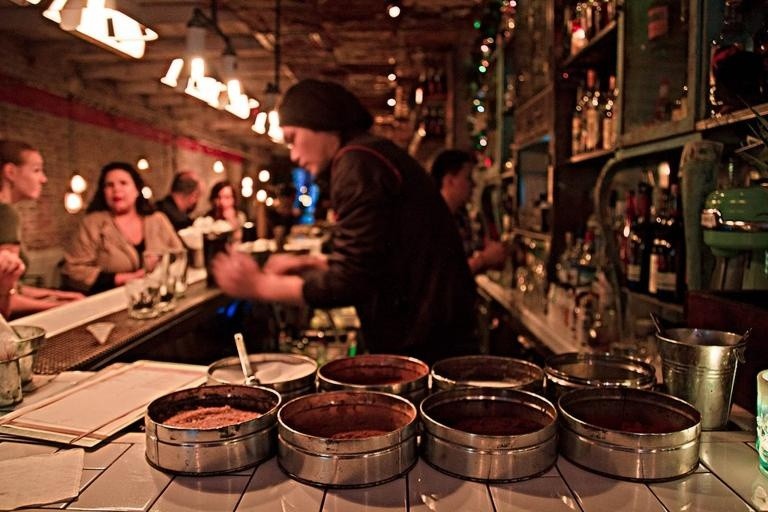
[754,370,768,476]
[655,326,742,427]
[125,245,188,322]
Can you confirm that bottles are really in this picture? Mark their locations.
[704,2,767,122]
[394,25,509,169]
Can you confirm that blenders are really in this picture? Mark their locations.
[700,188,768,294]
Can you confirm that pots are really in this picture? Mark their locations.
[276,390,419,488]
[515,5,677,342]
[418,387,559,486]
[557,385,701,486]
[205,354,318,399]
[143,385,282,476]
[426,355,544,396]
[542,353,656,400]
[314,354,429,405]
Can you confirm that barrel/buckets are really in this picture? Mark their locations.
[8,324,46,382]
[650,311,753,432]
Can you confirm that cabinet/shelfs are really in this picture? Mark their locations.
[463,43,503,282]
[504,2,554,291]
[555,2,621,167]
[617,1,700,150]
[692,1,768,131]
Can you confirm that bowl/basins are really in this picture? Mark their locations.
[1,322,46,410]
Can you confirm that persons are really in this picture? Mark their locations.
[429,150,506,273]
[209,78,481,371]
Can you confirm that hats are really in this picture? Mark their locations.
[278,79,375,133]
